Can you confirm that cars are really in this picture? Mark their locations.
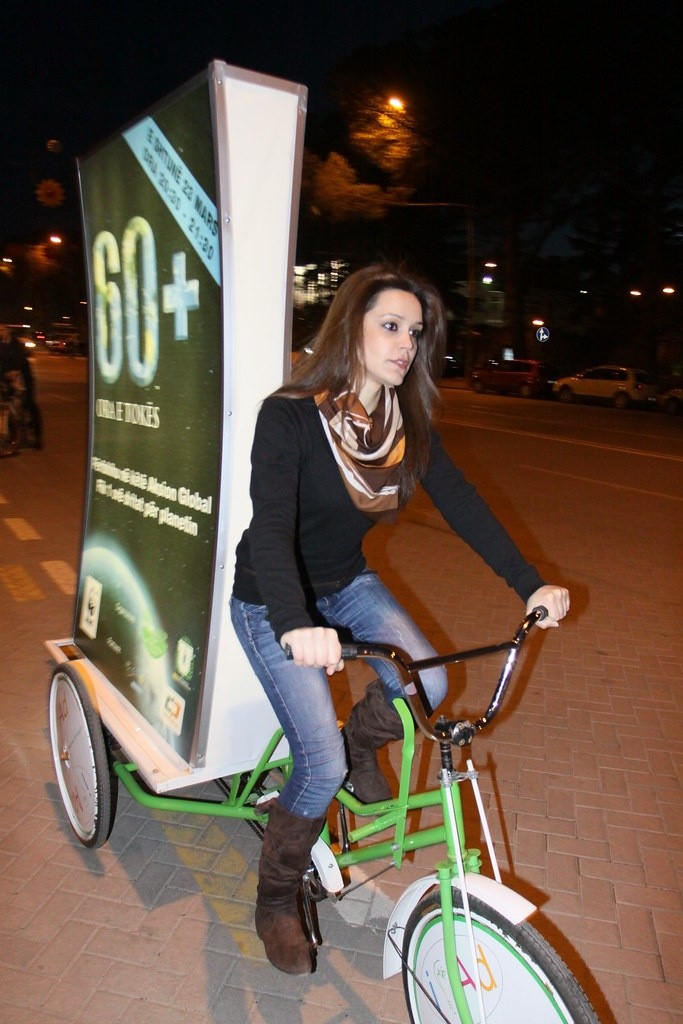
[0,323,85,354]
[656,379,683,417]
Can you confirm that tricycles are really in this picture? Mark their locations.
[47,601,607,1024]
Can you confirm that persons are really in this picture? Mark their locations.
[6,337,47,447]
[229,264,571,978]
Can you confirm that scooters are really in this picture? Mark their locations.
[1,368,38,459]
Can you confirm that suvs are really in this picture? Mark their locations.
[466,359,556,398]
[551,364,660,410]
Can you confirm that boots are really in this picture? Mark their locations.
[254,798,324,977]
[340,678,419,816]
